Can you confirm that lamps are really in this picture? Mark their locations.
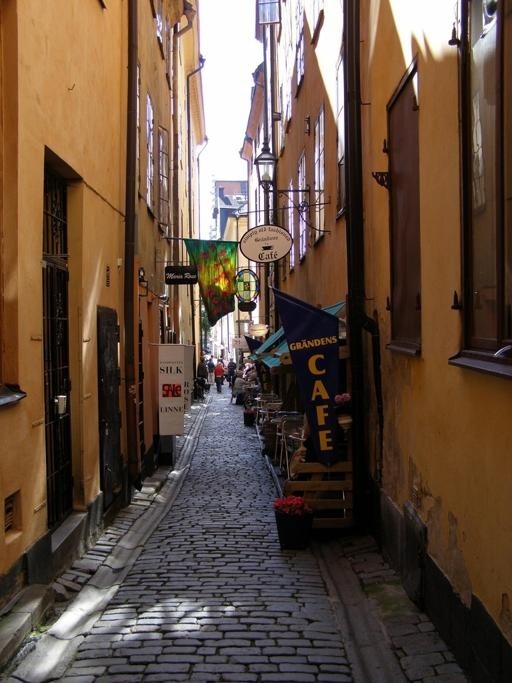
[255,141,310,194]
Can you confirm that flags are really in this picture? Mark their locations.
[183,238,238,328]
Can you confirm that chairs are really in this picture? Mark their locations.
[242,383,354,532]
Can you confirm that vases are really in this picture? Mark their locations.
[274,510,313,549]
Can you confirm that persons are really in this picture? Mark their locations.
[196,355,262,405]
